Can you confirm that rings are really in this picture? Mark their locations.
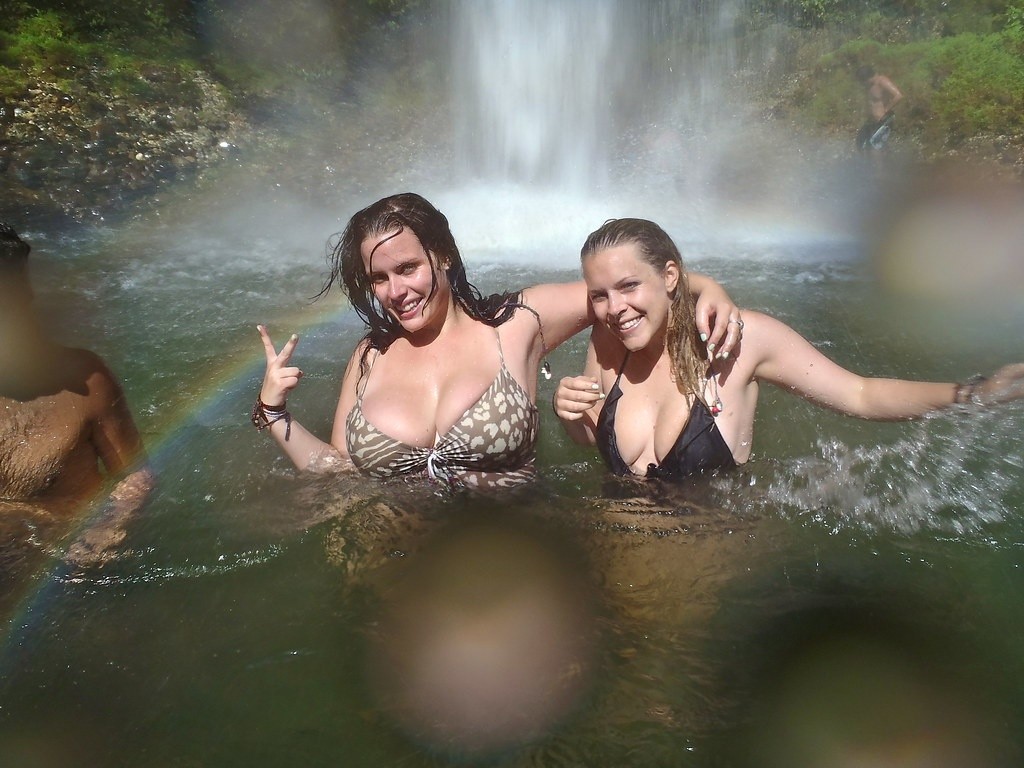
[729,320,744,330]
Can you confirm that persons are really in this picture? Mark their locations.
[552,219,1023,589]
[0,223,154,623]
[257,192,741,591]
[856,66,903,157]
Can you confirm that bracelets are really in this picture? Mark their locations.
[954,373,986,415]
[251,396,292,441]
